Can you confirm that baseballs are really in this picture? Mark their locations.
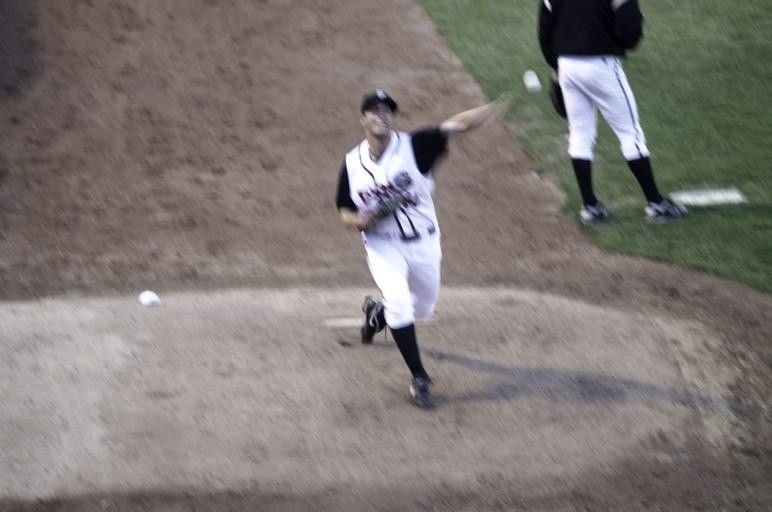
[138,290,162,308]
[522,69,541,93]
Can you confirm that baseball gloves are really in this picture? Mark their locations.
[362,192,403,220]
[549,77,568,119]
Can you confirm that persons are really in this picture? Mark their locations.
[536,1,691,228]
[333,89,514,410]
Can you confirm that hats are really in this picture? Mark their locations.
[360,88,398,117]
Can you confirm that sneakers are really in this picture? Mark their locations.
[644,196,690,226]
[406,374,436,409]
[359,295,385,345]
[578,201,615,223]
